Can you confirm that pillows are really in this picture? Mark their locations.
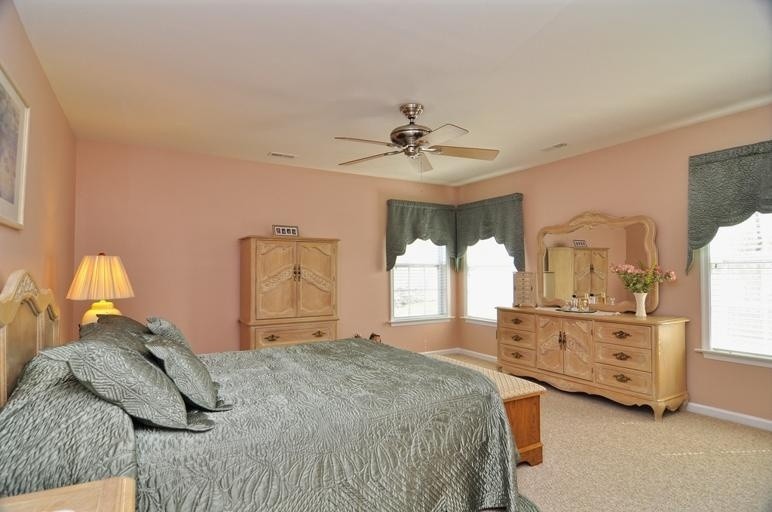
[38,314,234,433]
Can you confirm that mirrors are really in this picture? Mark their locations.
[537,210,659,315]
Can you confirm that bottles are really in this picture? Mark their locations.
[559,295,589,311]
[583,290,615,305]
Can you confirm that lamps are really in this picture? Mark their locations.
[65,253,135,325]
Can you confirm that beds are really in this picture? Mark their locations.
[0,269,521,512]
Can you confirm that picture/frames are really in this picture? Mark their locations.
[572,239,588,247]
[272,225,299,237]
[0,65,31,231]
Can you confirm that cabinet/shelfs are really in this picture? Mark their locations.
[546,246,611,304]
[494,306,691,421]
[237,235,341,352]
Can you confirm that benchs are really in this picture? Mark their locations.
[420,353,546,466]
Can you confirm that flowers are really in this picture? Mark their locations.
[608,259,676,293]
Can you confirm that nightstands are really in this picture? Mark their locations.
[0,477,136,512]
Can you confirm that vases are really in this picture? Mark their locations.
[632,292,648,317]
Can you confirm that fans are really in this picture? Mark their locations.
[334,103,500,174]
